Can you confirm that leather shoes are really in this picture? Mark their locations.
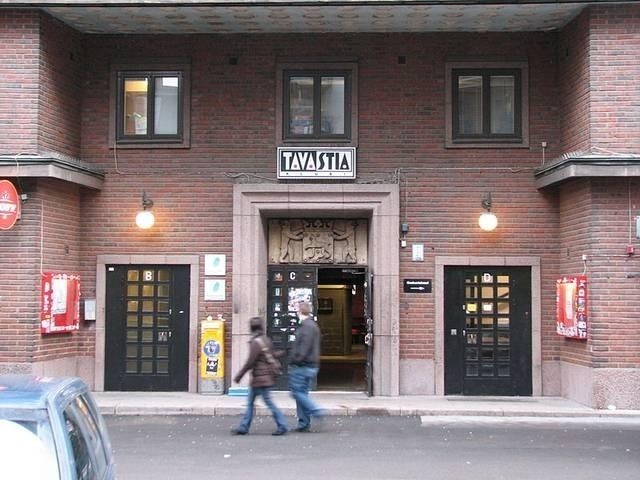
[231,428,247,435]
[271,423,311,436]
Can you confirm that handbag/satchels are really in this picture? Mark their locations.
[269,357,284,376]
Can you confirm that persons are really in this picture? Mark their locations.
[286,302,327,433]
[230,316,288,436]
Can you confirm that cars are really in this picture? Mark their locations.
[0,376,118,480]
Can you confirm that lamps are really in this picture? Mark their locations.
[134,187,155,231]
[476,190,499,234]
